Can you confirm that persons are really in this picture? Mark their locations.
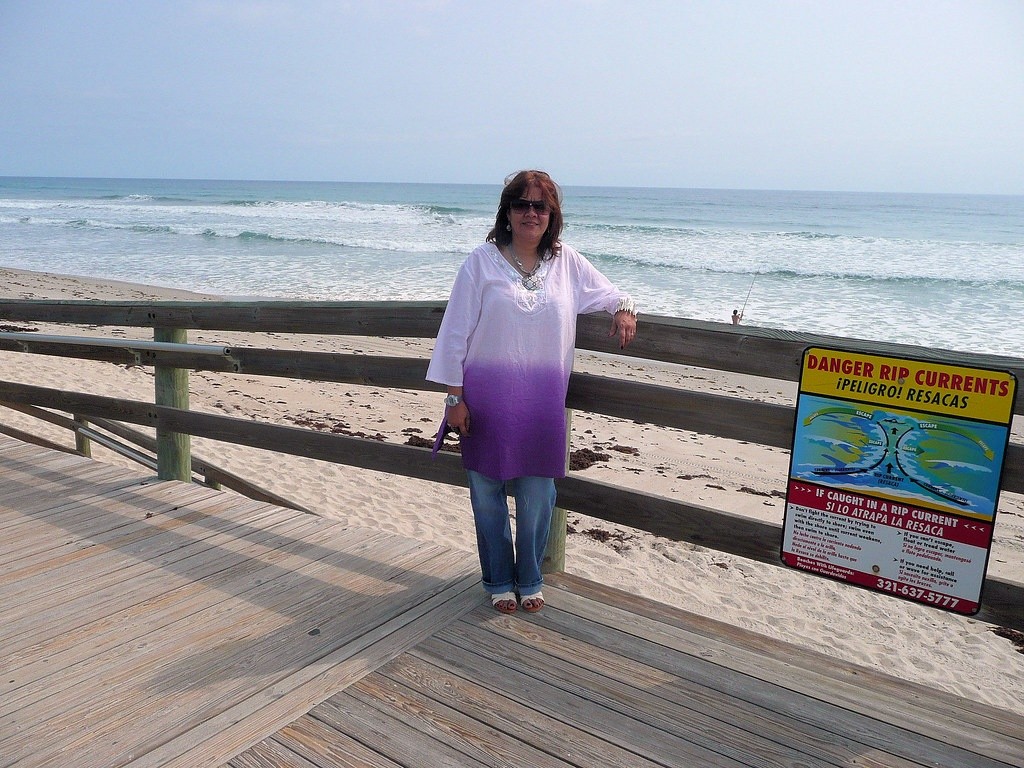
[732,310,741,325]
[426,171,637,613]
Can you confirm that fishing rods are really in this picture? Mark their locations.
[738,264,762,323]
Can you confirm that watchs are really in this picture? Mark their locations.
[444,395,463,406]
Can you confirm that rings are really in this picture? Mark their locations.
[631,333,634,335]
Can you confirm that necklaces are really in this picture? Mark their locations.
[508,244,541,289]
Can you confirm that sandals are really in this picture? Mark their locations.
[518,592,545,613]
[491,592,517,613]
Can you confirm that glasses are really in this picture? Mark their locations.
[511,199,553,214]
